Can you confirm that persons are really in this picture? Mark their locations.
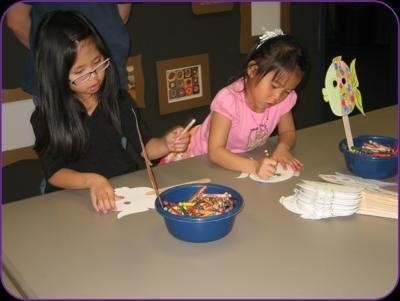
[6,2,132,195]
[27,10,191,214]
[158,28,304,180]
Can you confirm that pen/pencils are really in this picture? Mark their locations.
[177,118,197,139]
[162,185,234,216]
[351,139,398,157]
[263,148,271,157]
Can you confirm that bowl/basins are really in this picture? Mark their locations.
[339,134,399,179]
[153,183,245,244]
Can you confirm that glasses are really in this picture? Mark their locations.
[68,56,112,87]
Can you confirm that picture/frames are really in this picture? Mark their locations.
[128,53,212,116]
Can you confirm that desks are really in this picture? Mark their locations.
[1,102,399,300]
